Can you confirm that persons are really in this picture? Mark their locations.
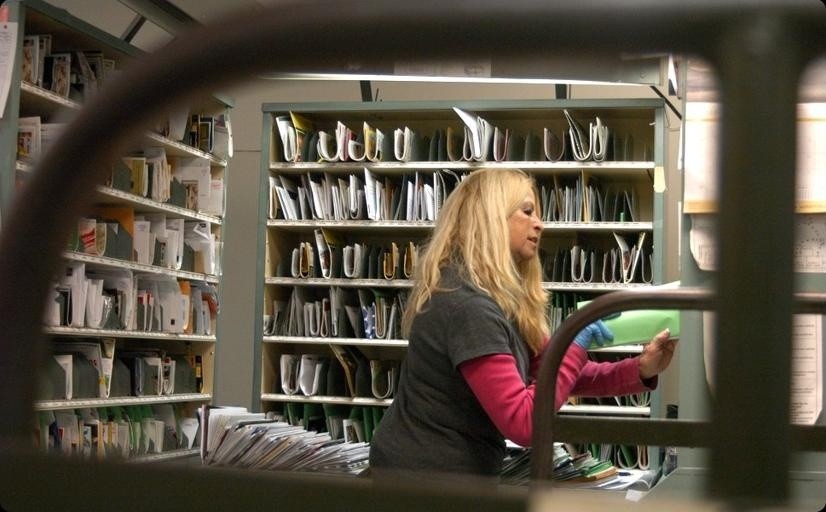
[370,168,675,476]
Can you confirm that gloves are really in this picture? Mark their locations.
[575,320,613,348]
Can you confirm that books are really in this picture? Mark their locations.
[33,338,206,461]
[500,437,680,489]
[275,106,609,163]
[263,227,431,442]
[537,231,656,409]
[17,36,118,167]
[102,103,234,219]
[43,206,224,335]
[269,168,635,222]
[195,404,370,476]
